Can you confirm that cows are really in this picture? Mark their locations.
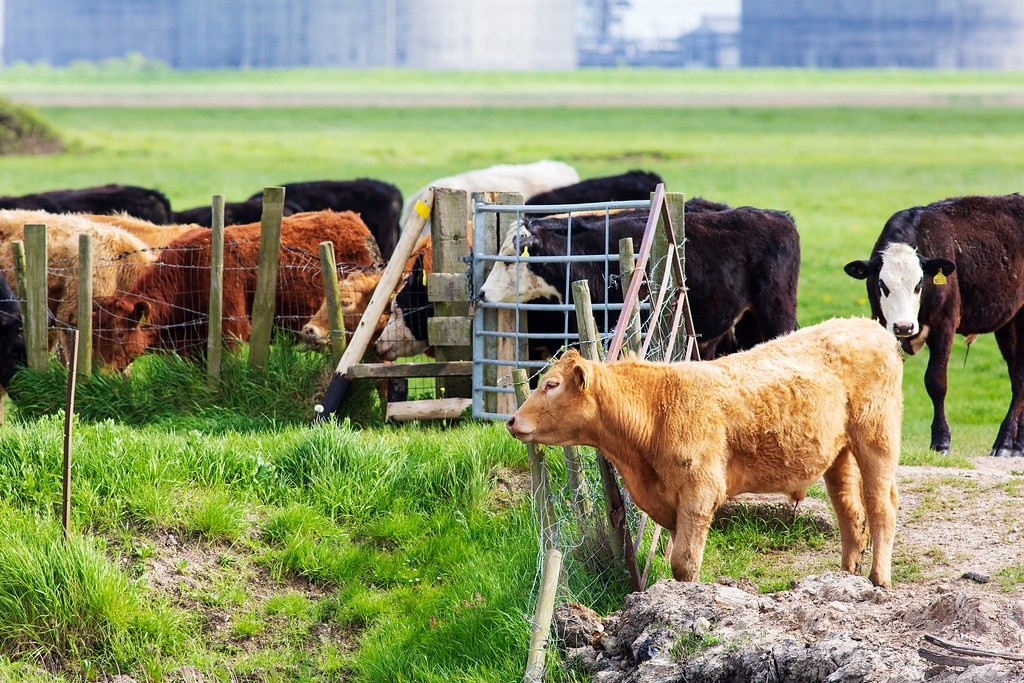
[507,312,906,590]
[844,191,1023,455]
[0,159,800,371]
